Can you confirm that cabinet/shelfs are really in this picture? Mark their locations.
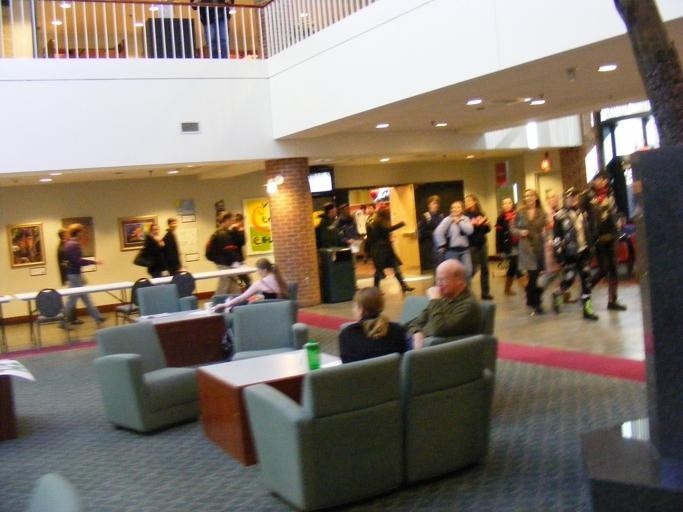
[143,18,196,59]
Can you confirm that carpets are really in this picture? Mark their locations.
[0,308,650,512]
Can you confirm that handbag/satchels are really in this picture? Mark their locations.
[134,248,155,267]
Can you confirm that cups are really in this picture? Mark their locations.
[205,302,213,313]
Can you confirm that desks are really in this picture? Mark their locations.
[0,295,12,353]
[142,267,258,288]
[20,281,133,347]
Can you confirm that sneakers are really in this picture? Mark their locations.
[607,302,627,309]
[58,315,107,330]
[482,294,493,299]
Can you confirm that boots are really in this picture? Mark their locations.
[395,272,414,292]
[374,276,380,287]
[552,285,578,313]
[504,276,545,316]
[581,294,599,319]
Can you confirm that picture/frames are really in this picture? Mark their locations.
[62,216,95,257]
[117,215,158,251]
[6,222,47,268]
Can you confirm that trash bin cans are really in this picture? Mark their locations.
[318,246,356,303]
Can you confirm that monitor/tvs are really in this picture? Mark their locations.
[307,166,335,198]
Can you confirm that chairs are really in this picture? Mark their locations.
[115,277,153,325]
[404,335,497,486]
[242,353,404,512]
[212,279,298,328]
[171,271,195,298]
[232,300,308,361]
[396,295,496,346]
[136,284,197,316]
[35,288,71,351]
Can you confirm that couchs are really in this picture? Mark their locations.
[93,302,199,434]
[249,281,302,321]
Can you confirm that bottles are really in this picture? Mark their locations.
[306,338,320,370]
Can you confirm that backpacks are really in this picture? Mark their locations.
[206,231,218,261]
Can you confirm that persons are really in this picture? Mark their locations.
[402,258,484,337]
[56,223,107,331]
[337,287,424,364]
[205,211,251,296]
[191,1,234,58]
[145,217,181,278]
[417,172,645,320]
[315,202,414,304]
[209,258,290,314]
